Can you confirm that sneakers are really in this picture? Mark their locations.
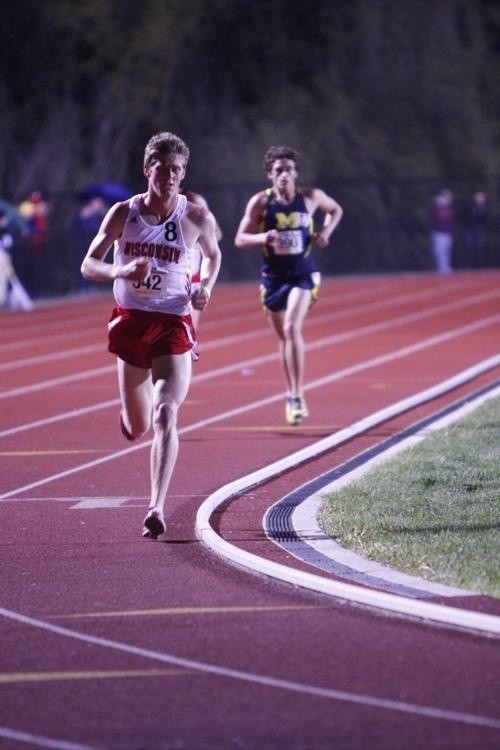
[141,506,166,542]
[285,395,310,426]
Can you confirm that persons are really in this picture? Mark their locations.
[236,146,345,428]
[460,189,490,270]
[177,186,223,363]
[0,180,136,310]
[78,131,224,540]
[430,188,459,275]
